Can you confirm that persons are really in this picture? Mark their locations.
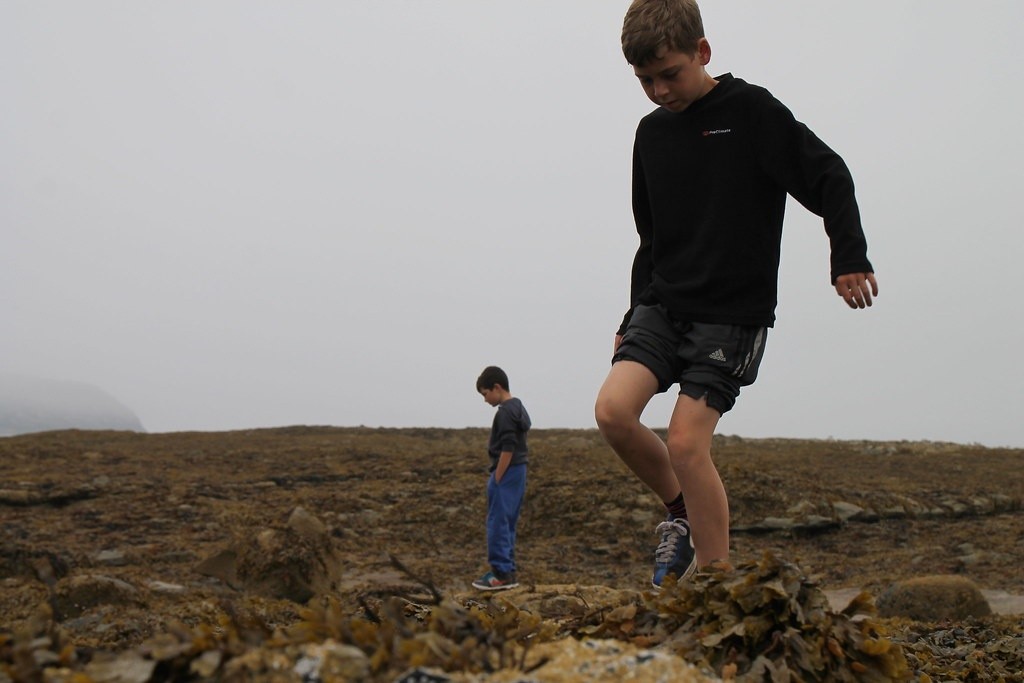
[593,0,880,587]
[472,366,531,590]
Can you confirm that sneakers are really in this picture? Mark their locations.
[652,513,697,589]
[472,570,519,589]
